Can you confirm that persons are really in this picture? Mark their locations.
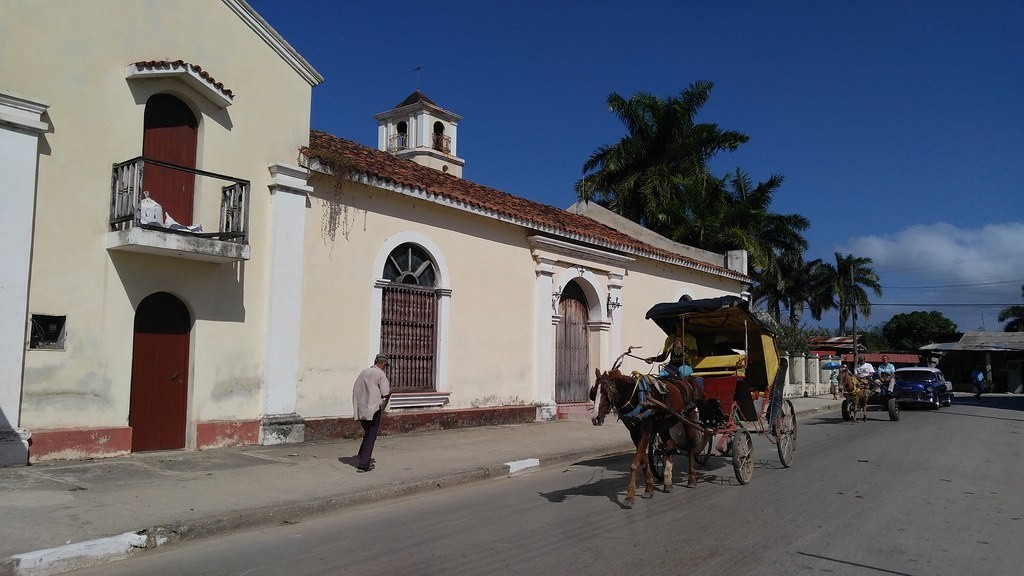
[352,354,392,472]
[646,318,698,379]
[971,364,985,401]
[874,367,878,375]
[871,355,895,394]
[829,369,838,400]
[854,356,875,381]
[702,334,740,371]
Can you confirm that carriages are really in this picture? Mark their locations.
[836,367,900,421]
[591,295,798,510]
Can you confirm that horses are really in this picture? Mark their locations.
[590,367,705,510]
[838,368,869,423]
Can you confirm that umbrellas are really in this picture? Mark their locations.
[822,362,842,374]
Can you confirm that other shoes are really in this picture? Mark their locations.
[888,393,892,400]
[358,464,375,473]
[370,458,376,463]
[976,396,982,402]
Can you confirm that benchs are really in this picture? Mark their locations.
[690,355,744,376]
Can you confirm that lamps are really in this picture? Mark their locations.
[551,286,567,315]
[607,293,622,318]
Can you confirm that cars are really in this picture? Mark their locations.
[895,366,953,410]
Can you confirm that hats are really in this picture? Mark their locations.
[858,356,864,361]
[374,354,390,366]
[976,364,981,369]
